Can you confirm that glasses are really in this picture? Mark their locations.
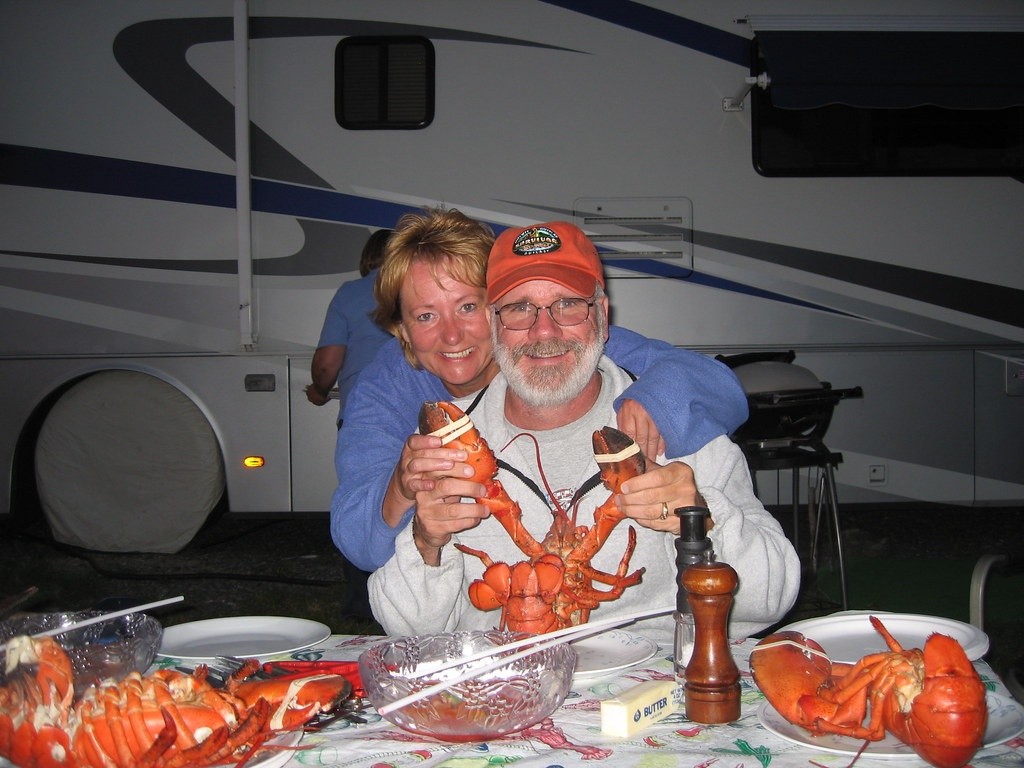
[496,297,594,331]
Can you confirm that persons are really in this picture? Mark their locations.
[367,222,800,640]
[306,229,395,431]
[329,206,752,574]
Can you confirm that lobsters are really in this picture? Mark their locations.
[747,616,988,768]
[420,401,646,635]
[0,634,352,768]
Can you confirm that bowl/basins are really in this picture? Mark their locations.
[356,630,577,740]
[1,607,164,703]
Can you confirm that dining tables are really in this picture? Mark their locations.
[0,635,1024,768]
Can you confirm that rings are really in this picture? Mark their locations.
[661,502,668,519]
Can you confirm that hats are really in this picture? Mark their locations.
[486,221,606,306]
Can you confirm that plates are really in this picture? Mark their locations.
[757,681,1024,756]
[152,616,333,659]
[765,611,991,670]
[567,627,659,680]
